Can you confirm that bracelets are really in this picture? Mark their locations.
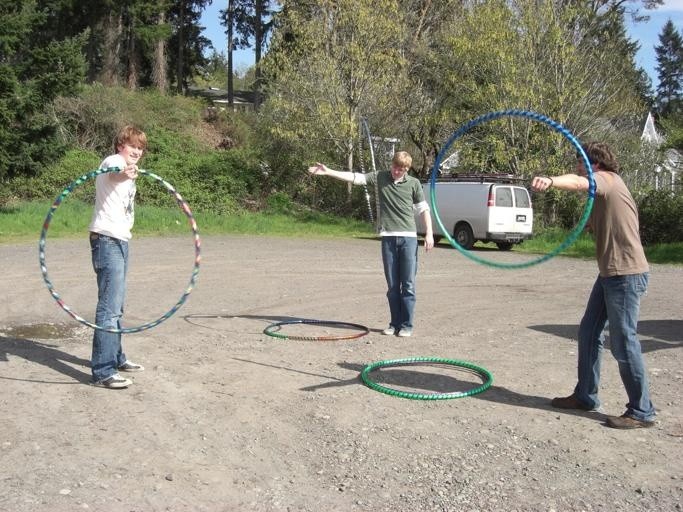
[545,176,553,191]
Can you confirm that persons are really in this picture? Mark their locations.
[307,150,435,337]
[87,124,147,389]
[531,141,657,429]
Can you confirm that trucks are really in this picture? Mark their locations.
[410,174,532,250]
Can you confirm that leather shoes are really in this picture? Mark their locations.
[606,415,655,430]
[551,396,582,409]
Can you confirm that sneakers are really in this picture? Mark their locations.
[397,330,410,337]
[94,374,132,388]
[382,328,398,335]
[118,360,144,372]
